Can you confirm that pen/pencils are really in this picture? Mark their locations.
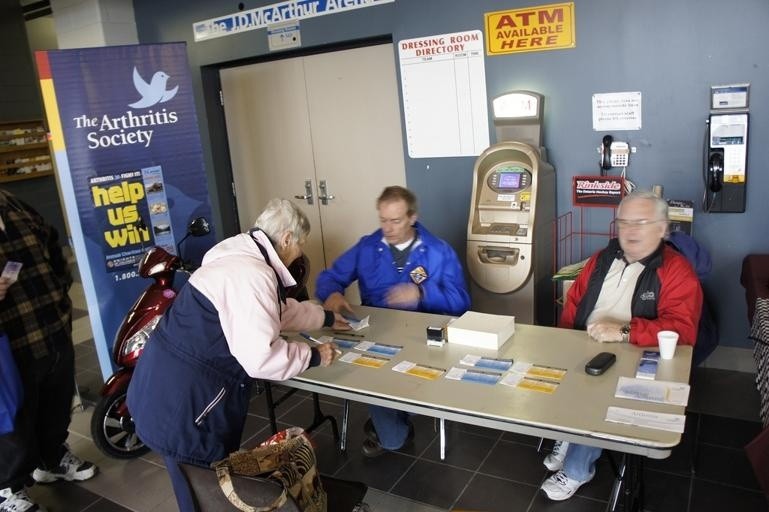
[299,332,343,355]
[334,332,365,337]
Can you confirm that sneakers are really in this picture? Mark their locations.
[31,442,98,484]
[0,488,48,512]
[360,412,415,459]
[538,438,596,503]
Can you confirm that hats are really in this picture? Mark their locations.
[666,230,714,284]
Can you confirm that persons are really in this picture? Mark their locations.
[537,190,705,504]
[125,196,354,512]
[1,190,100,486]
[313,183,472,458]
[1,273,47,511]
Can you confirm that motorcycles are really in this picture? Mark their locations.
[89,215,312,462]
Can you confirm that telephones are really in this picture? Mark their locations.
[600,135,629,170]
[711,153,723,193]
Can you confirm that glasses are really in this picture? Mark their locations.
[615,217,665,229]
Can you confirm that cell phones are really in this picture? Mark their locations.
[587,352,615,375]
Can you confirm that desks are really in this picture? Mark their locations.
[253,302,698,512]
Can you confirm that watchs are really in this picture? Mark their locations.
[619,321,631,343]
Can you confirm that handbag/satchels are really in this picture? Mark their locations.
[210,425,327,511]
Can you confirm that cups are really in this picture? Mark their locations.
[657,331,680,360]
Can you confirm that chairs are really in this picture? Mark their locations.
[536,282,722,512]
[333,233,465,465]
